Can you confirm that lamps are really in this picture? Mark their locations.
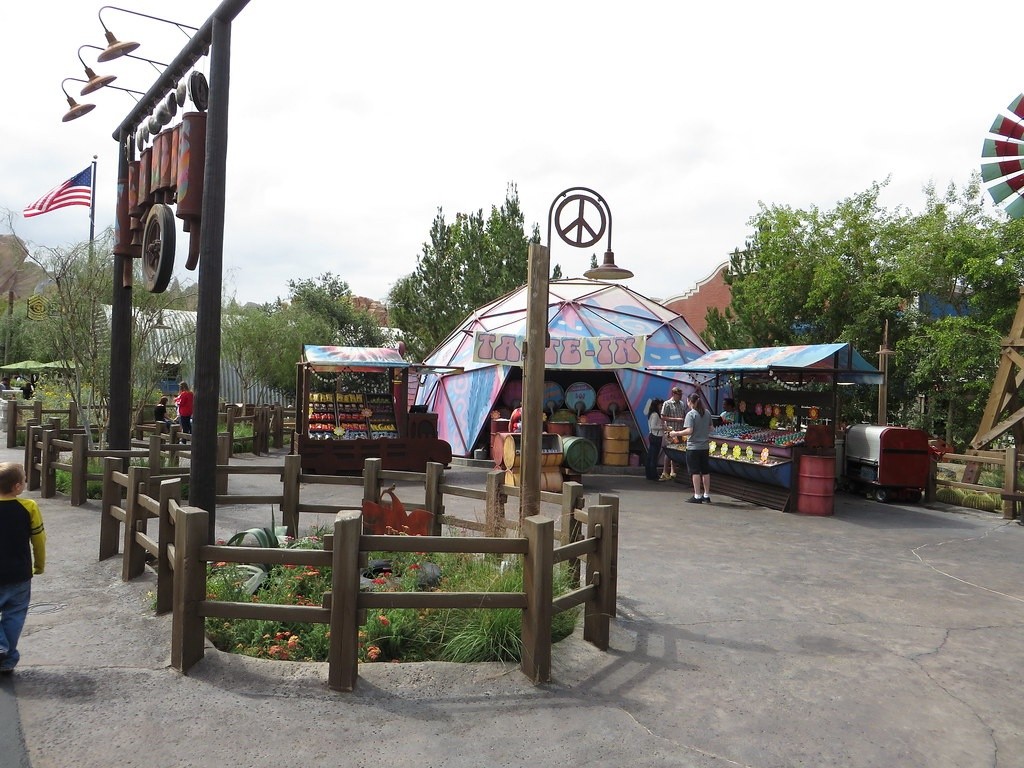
[61,78,146,122]
[97,5,200,62]
[78,45,168,96]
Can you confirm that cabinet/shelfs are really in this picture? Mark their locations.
[308,391,398,439]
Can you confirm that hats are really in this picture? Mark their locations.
[672,387,683,395]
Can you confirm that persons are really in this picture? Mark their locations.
[670,393,714,504]
[154,381,194,444]
[508,406,522,433]
[720,398,739,424]
[0,374,36,400]
[645,397,669,482]
[660,386,686,479]
[0,462,47,673]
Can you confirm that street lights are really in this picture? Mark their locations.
[520,185,635,536]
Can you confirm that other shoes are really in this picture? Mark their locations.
[660,472,672,480]
[703,496,711,503]
[653,476,664,481]
[0,664,12,671]
[670,472,677,477]
[687,495,702,504]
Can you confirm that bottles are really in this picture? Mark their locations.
[672,435,678,443]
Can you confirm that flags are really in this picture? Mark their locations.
[24,165,91,218]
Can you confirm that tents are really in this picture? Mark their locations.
[414,278,734,465]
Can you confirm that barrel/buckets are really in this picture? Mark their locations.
[834,439,845,483]
[798,454,837,516]
[474,379,639,493]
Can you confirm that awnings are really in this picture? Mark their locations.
[0,360,83,372]
[646,343,884,448]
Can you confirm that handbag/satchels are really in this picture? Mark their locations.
[649,433,659,443]
